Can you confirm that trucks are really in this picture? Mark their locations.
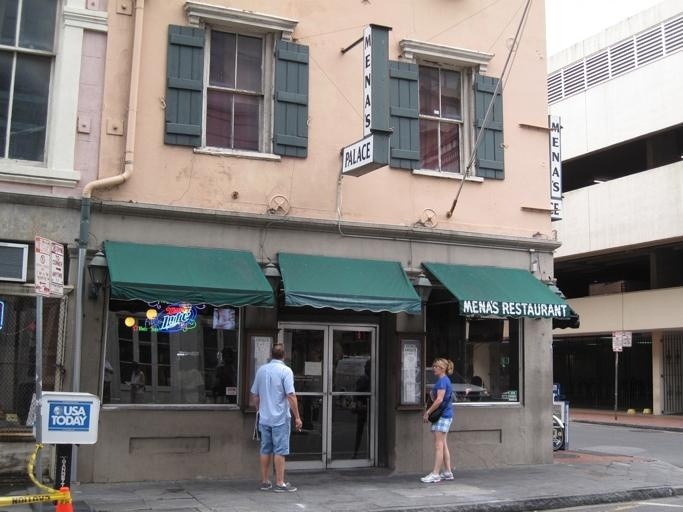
[331,353,370,412]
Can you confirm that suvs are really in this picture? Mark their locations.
[424,365,488,403]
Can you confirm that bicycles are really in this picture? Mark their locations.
[552,414,566,452]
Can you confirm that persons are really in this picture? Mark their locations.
[251,343,303,492]
[352,360,371,459]
[420,358,454,483]
[212,348,236,404]
[124,362,146,404]
[170,355,206,404]
[102,358,114,405]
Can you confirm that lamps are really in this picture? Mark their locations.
[260,255,282,310]
[85,247,107,300]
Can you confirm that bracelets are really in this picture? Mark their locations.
[427,409,430,416]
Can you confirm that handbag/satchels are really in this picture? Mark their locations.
[425,398,450,424]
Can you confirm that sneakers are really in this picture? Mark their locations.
[439,472,454,481]
[274,483,297,493]
[419,473,440,483]
[259,481,272,491]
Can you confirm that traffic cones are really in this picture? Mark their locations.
[53,486,75,512]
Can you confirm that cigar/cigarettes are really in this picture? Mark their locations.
[297,428,302,433]
